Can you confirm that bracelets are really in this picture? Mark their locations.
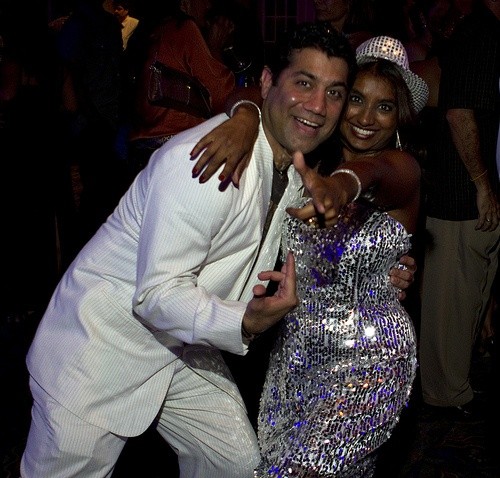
[330,168,361,204]
[229,100,262,123]
[240,322,261,343]
[471,169,489,182]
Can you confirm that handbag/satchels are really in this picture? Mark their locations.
[144,34,212,120]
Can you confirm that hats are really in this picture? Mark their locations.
[355,35,429,114]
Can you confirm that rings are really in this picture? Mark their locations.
[486,219,492,222]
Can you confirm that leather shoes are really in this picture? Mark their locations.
[423,401,489,425]
[466,376,491,395]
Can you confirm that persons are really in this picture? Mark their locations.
[0,0,450,199]
[415,1,500,427]
[189,36,431,478]
[19,21,420,478]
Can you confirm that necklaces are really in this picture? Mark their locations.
[273,162,289,178]
